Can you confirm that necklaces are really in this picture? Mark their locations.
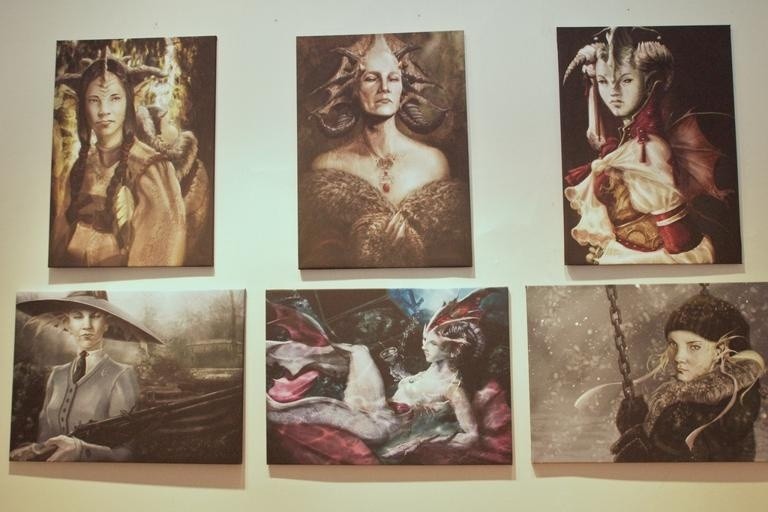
[363,126,402,192]
[408,365,434,384]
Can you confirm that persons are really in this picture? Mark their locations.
[49,49,187,267]
[298,35,470,266]
[607,288,766,462]
[15,291,164,463]
[561,27,736,264]
[266,296,482,463]
